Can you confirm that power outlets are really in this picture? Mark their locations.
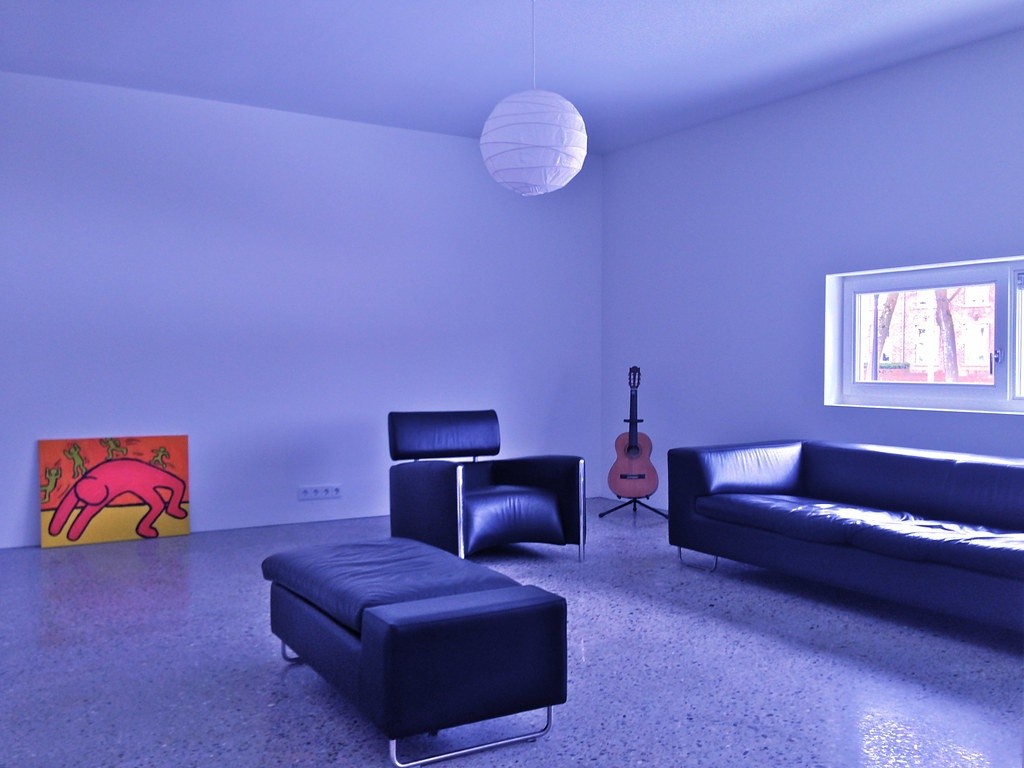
[297,483,343,501]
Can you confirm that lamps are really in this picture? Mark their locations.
[479,0,590,200]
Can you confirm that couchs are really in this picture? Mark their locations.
[260,534,574,766]
[386,405,589,562]
[667,439,1023,641]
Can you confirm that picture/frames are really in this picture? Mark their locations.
[34,433,194,543]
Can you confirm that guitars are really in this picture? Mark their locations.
[607,366,659,500]
